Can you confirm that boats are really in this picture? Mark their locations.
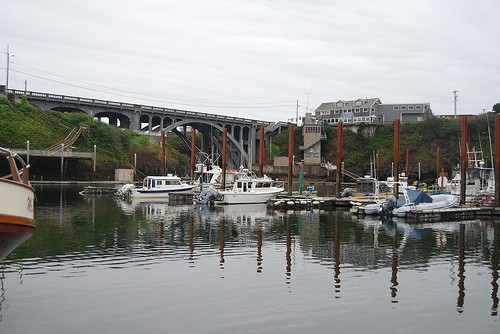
[0,223,35,263]
[122,173,197,198]
[193,169,286,204]
[396,192,458,217]
[0,147,37,229]
